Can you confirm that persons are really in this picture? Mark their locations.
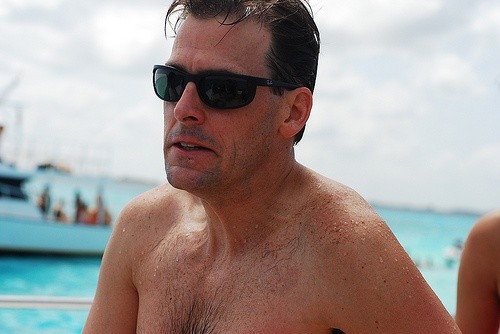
[87,1,461,333]
[457,208,500,333]
[38,185,115,226]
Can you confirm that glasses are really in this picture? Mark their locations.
[152,63,302,109]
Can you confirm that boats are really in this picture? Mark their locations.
[1,195,116,254]
[0,160,32,184]
